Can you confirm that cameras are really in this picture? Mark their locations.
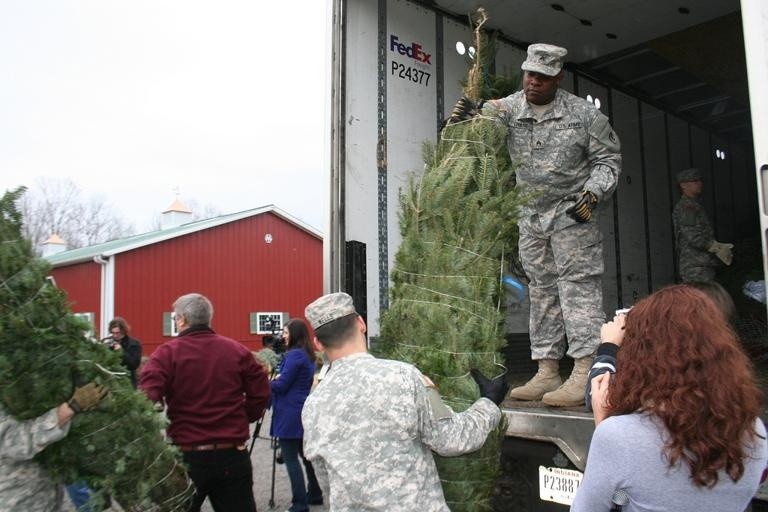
[263,317,287,355]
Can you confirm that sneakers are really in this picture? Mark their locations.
[283,506,312,512]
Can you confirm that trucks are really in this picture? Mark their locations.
[323,1,768,506]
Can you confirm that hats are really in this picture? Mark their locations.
[304,292,355,330]
[522,43,567,77]
[676,169,702,183]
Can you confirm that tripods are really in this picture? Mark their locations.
[248,363,290,508]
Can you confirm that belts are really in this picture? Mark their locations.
[182,441,234,451]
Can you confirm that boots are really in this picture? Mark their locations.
[542,356,593,408]
[510,361,563,401]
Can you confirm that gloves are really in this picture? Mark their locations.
[69,381,108,412]
[564,190,597,224]
[469,363,510,405]
[452,95,483,121]
[709,240,734,266]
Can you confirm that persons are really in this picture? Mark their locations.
[572,282,768,512]
[671,167,733,289]
[586,280,734,426]
[301,292,511,512]
[0,383,107,512]
[471,42,622,408]
[100,318,142,389]
[268,317,324,511]
[136,294,270,512]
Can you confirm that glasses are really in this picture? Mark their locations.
[110,331,122,335]
